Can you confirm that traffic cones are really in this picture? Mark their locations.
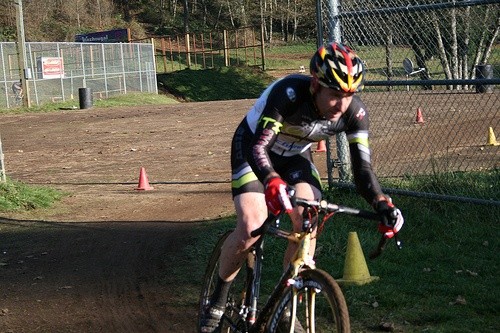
[481,126,500,146]
[334,231,380,287]
[314,139,326,153]
[134,165,152,191]
[414,107,426,124]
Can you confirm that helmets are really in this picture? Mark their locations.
[308,43,366,94]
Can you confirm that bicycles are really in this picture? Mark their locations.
[197,187,404,333]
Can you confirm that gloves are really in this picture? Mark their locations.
[262,176,297,217]
[376,197,404,239]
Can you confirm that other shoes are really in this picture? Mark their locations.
[279,308,305,333]
[200,302,226,333]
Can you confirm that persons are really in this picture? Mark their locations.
[199,44,404,332]
